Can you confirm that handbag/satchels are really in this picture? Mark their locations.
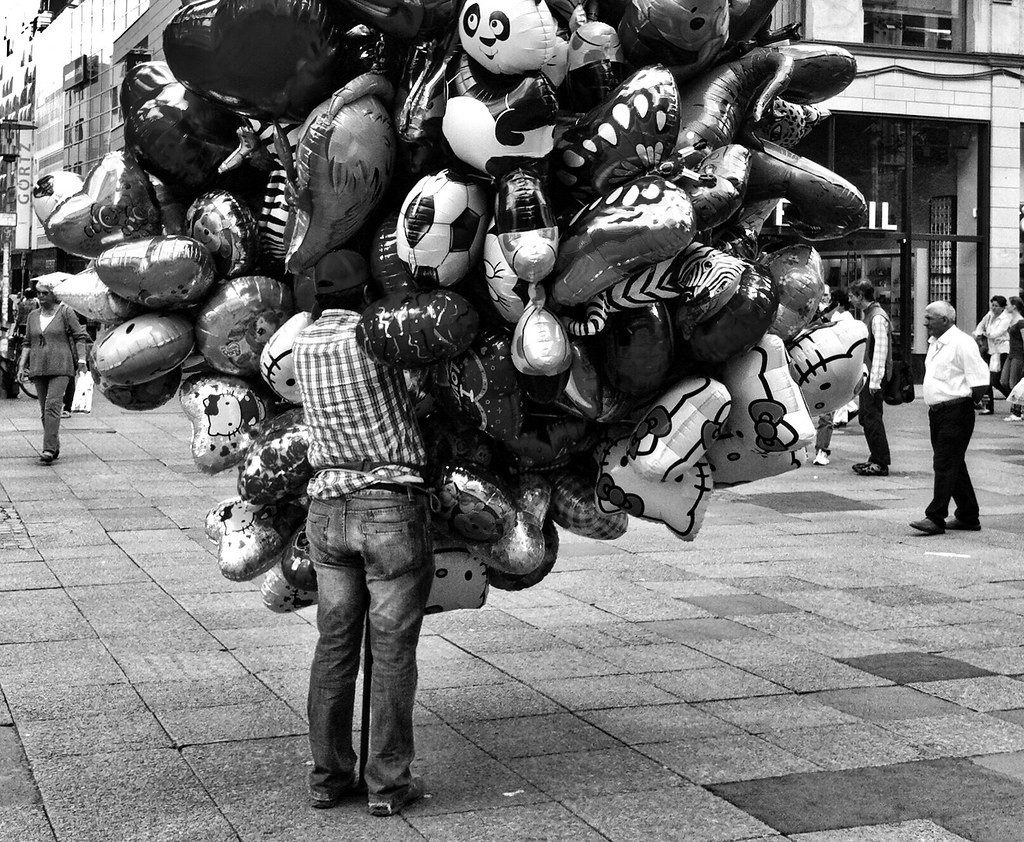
[63,306,91,370]
[975,333,988,356]
[1006,378,1024,405]
[882,360,914,406]
[71,372,93,413]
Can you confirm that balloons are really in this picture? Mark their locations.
[33,0,873,615]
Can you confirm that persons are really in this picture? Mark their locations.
[909,300,990,533]
[847,278,891,476]
[975,289,1024,423]
[813,283,835,466]
[17,283,93,463]
[61,308,92,417]
[290,249,428,818]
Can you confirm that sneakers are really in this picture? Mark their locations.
[368,773,424,817]
[811,450,829,464]
[858,464,888,475]
[851,460,876,471]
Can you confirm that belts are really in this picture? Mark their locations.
[370,483,424,495]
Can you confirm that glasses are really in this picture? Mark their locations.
[35,290,52,296]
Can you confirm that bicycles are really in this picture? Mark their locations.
[16,355,38,399]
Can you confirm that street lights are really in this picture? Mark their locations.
[0,119,38,323]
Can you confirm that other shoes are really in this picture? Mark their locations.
[1002,414,1023,422]
[310,798,337,809]
[910,517,945,534]
[39,451,54,461]
[943,519,982,531]
[980,409,993,416]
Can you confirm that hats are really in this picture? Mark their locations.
[312,248,369,294]
